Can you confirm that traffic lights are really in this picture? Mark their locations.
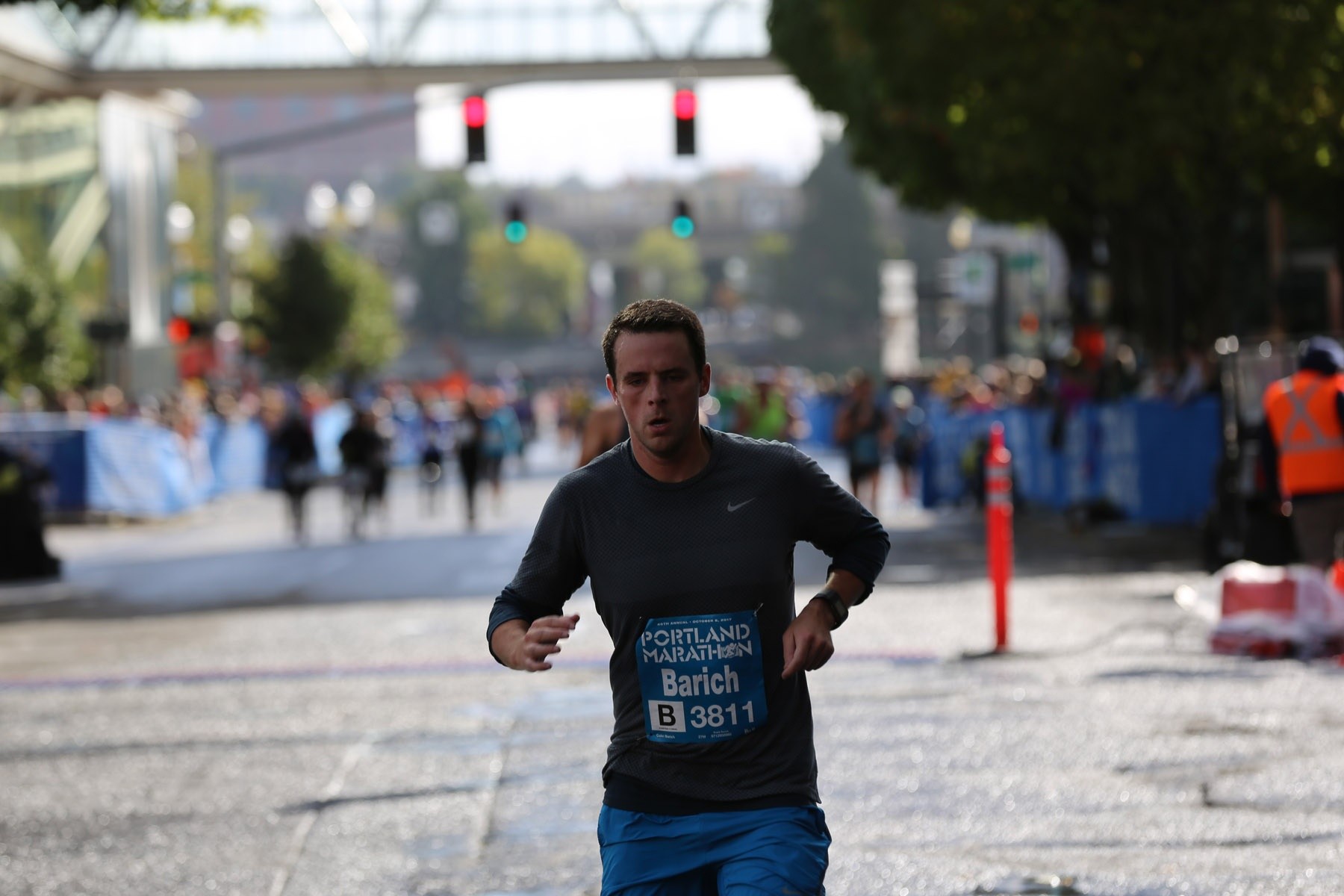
[466,96,488,162]
[164,318,189,341]
[673,202,697,239]
[505,203,524,244]
[675,88,697,154]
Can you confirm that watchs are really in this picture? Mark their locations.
[809,589,849,630]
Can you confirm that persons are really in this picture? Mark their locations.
[487,299,891,896]
[699,334,1344,571]
[52,369,629,545]
